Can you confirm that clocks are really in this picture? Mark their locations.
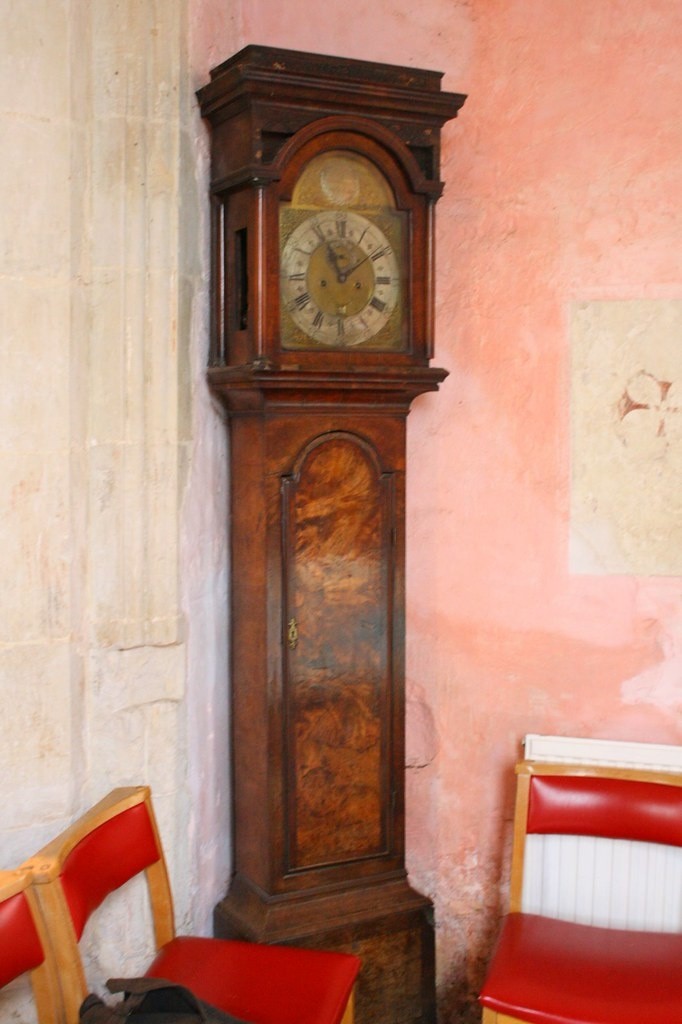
[195,43,442,1023]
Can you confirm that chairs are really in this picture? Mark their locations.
[17,784,365,1023]
[3,874,68,1023]
[474,733,682,1023]
